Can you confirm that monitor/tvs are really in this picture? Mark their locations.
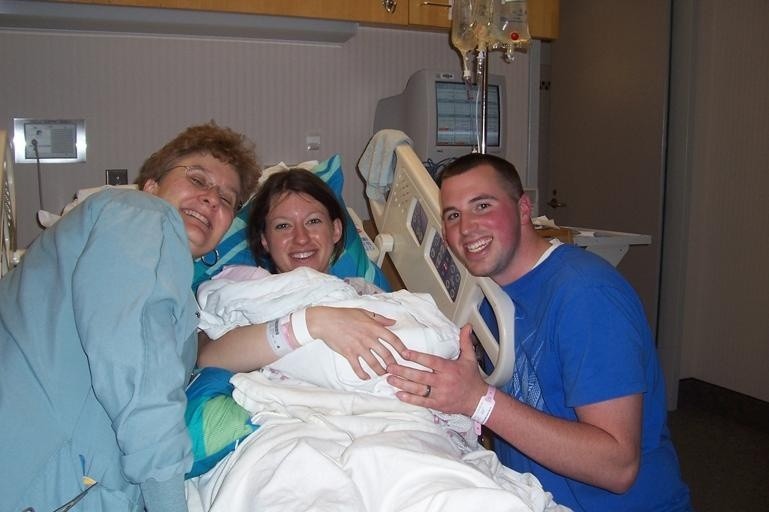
[373,70,506,167]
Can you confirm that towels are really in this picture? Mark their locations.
[354,130,415,203]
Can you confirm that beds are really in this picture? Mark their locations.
[11,129,576,512]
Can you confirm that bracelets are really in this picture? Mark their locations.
[266,311,300,358]
[469,385,499,438]
[291,307,315,346]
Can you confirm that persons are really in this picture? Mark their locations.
[0,120,264,512]
[195,165,409,381]
[384,151,694,512]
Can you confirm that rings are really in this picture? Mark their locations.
[370,311,377,322]
[422,385,432,397]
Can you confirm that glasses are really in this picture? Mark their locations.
[167,165,238,208]
